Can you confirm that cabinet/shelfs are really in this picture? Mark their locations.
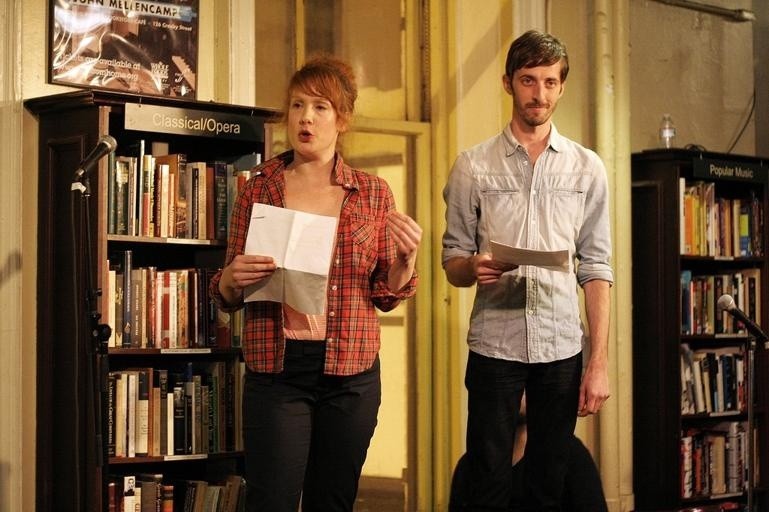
[23,90,285,512]
[632,149,769,512]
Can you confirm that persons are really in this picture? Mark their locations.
[206,49,422,511]
[440,29,615,510]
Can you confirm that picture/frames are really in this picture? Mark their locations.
[48,0,199,100]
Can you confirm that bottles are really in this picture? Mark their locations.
[660,112,676,150]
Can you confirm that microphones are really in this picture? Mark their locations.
[75,135,118,178]
[718,294,769,345]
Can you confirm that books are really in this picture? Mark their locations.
[108,355,246,457]
[106,139,262,242]
[106,250,244,349]
[108,471,246,511]
[677,177,765,511]
